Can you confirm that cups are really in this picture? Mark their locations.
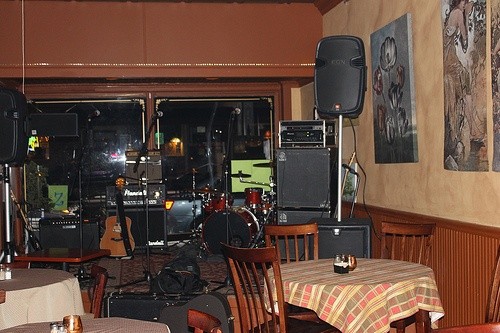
[333,255,349,274]
[62,314,83,333]
[346,255,357,271]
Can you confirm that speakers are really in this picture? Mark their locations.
[107,209,168,250]
[124,150,163,184]
[38,217,98,250]
[313,36,368,118]
[166,194,207,234]
[0,90,29,167]
[274,148,371,260]
[28,112,78,138]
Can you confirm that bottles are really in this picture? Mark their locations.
[50,322,66,333]
[0,264,12,281]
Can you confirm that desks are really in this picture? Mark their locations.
[105,209,168,249]
[0,268,85,331]
[0,316,171,333]
[11,249,110,274]
[263,257,444,333]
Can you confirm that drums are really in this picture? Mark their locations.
[204,191,234,213]
[244,187,263,209]
[252,194,274,215]
[202,206,260,254]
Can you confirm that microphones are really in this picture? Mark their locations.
[231,108,240,115]
[88,110,100,117]
[153,111,163,117]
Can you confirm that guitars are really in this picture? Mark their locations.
[99,177,135,257]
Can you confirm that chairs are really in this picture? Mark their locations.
[264,222,319,323]
[186,309,221,333]
[379,222,437,333]
[87,266,108,320]
[429,254,500,333]
[218,242,327,333]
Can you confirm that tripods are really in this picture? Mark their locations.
[0,164,18,264]
[114,117,158,290]
[210,114,264,293]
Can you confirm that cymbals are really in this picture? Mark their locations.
[253,162,270,167]
[230,174,252,177]
